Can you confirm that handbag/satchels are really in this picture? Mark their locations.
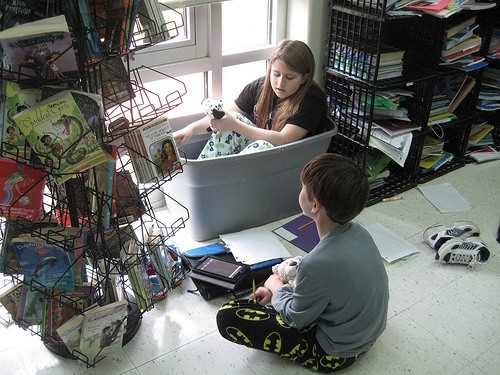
[181,241,284,301]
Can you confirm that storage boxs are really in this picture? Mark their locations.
[164,117,338,242]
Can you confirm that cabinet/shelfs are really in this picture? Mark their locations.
[324,0,500,207]
[0,0,190,369]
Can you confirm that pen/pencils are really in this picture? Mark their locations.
[382,196,403,201]
[253,279,256,303]
[296,220,314,231]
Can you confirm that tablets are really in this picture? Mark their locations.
[192,254,250,284]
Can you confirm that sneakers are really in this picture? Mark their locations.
[423,221,480,250]
[435,237,490,270]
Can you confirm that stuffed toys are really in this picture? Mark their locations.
[272,256,302,293]
[201,98,225,134]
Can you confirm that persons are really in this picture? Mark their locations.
[171,38,330,161]
[215,153,391,373]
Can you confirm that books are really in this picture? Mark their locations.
[329,0,500,191]
[0,0,186,366]
